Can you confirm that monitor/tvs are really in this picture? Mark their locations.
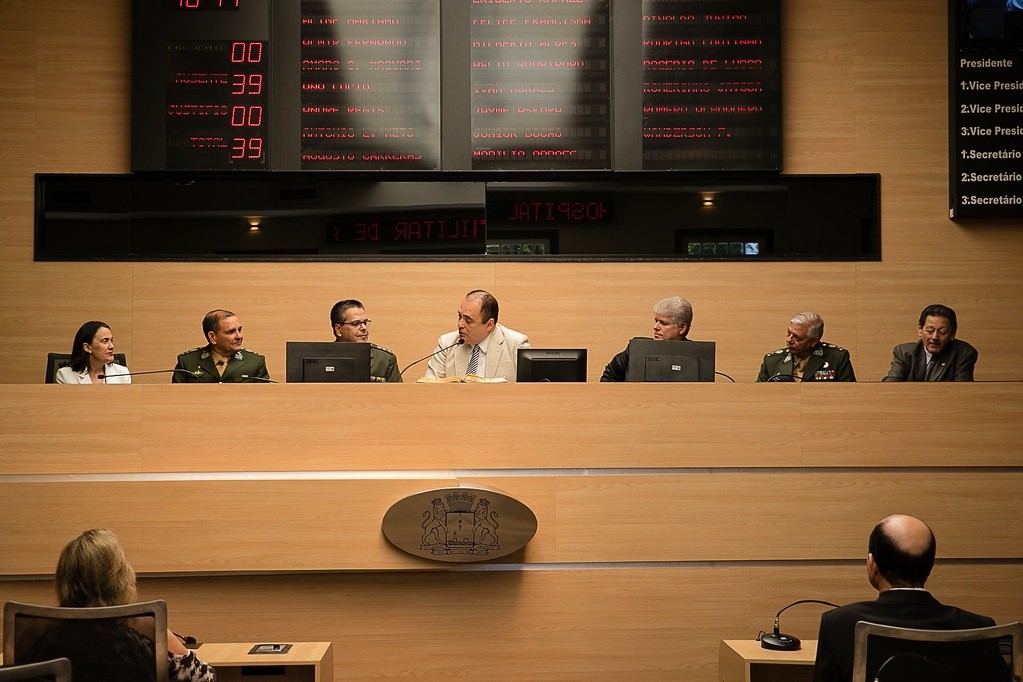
[286,342,371,382]
[627,339,716,383]
[516,349,587,382]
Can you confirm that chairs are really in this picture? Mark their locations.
[0,598,169,682]
[853,620,1023,682]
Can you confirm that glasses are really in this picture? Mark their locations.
[335,320,374,328]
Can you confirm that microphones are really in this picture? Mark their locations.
[173,632,196,645]
[767,374,815,382]
[98,369,205,384]
[400,339,465,375]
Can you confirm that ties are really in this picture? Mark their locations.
[466,345,481,376]
[219,357,229,377]
[924,355,940,381]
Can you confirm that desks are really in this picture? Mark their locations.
[184,641,333,682]
[718,639,819,682]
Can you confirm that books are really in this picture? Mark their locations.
[416,374,507,383]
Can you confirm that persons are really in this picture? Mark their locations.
[55,320,131,385]
[171,309,269,383]
[814,514,1012,682]
[599,296,692,382]
[20,528,216,682]
[755,313,856,382]
[424,290,531,382]
[330,299,403,383]
[881,304,978,383]
